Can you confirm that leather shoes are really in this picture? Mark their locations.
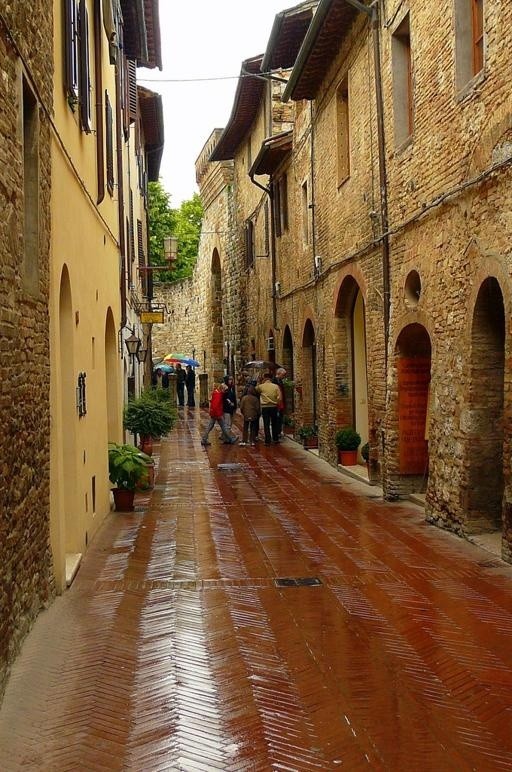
[264,443,269,446]
[273,441,279,444]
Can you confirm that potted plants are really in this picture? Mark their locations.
[283,415,295,434]
[294,424,318,449]
[107,386,178,513]
[336,428,370,479]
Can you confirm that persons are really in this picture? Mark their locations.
[154,364,195,406]
[201,368,287,446]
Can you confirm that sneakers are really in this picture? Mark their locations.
[224,436,239,444]
[251,441,255,445]
[238,441,246,446]
[201,440,211,445]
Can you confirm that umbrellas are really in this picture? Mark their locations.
[242,361,283,382]
[152,354,200,373]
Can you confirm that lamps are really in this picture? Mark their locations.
[136,233,180,271]
[123,326,147,363]
